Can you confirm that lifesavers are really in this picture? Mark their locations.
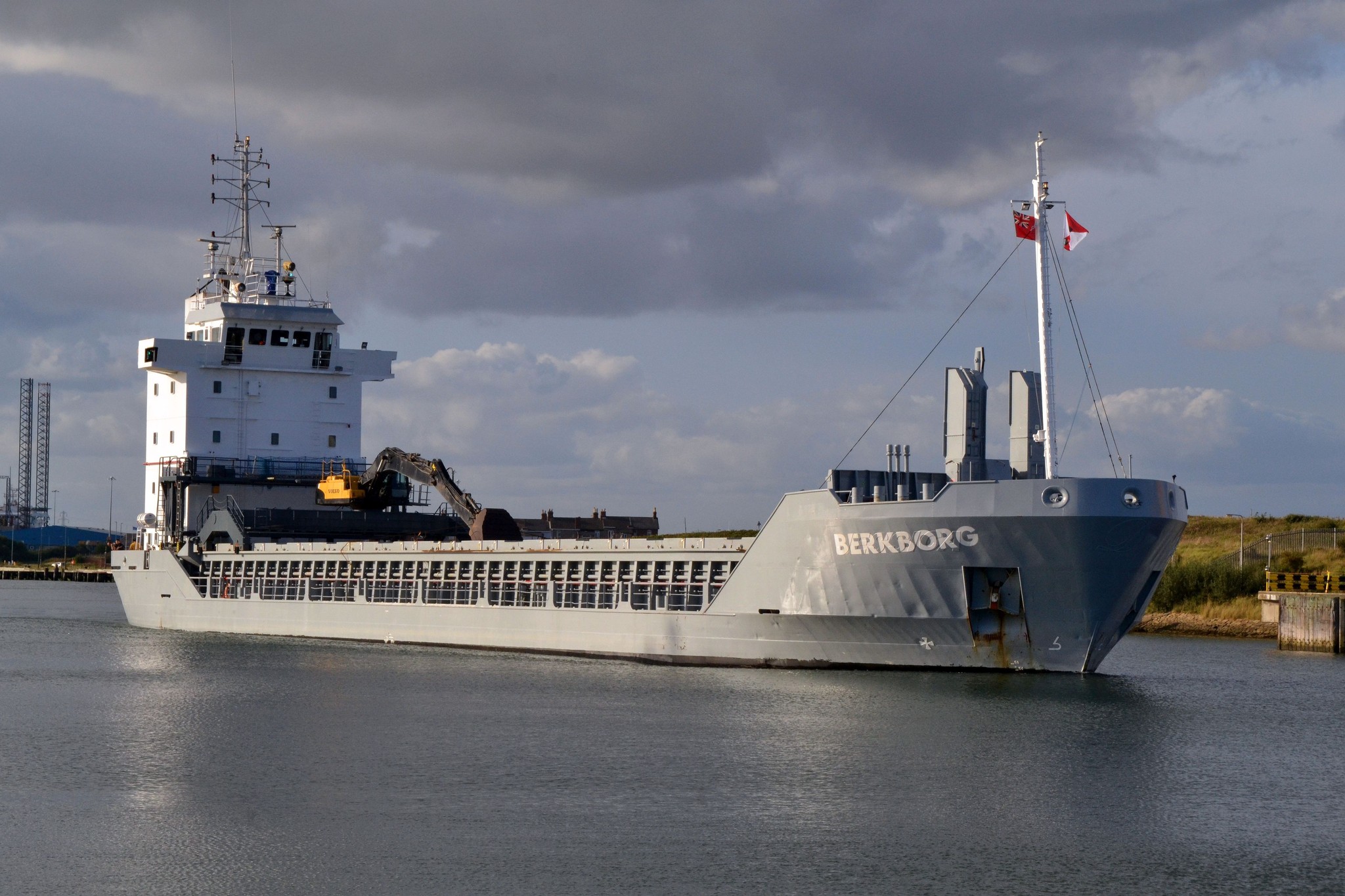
[224,583,231,599]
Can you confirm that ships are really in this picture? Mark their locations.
[105,77,1204,677]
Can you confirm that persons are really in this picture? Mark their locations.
[110,540,125,550]
[128,538,143,550]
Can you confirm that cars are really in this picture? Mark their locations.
[51,562,64,568]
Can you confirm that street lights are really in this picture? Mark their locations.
[120,523,123,539]
[52,489,61,526]
[12,525,15,566]
[115,522,118,540]
[107,476,116,540]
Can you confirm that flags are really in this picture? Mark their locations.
[1062,210,1090,252]
[1013,209,1036,241]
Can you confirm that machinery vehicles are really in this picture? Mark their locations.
[313,444,526,539]
